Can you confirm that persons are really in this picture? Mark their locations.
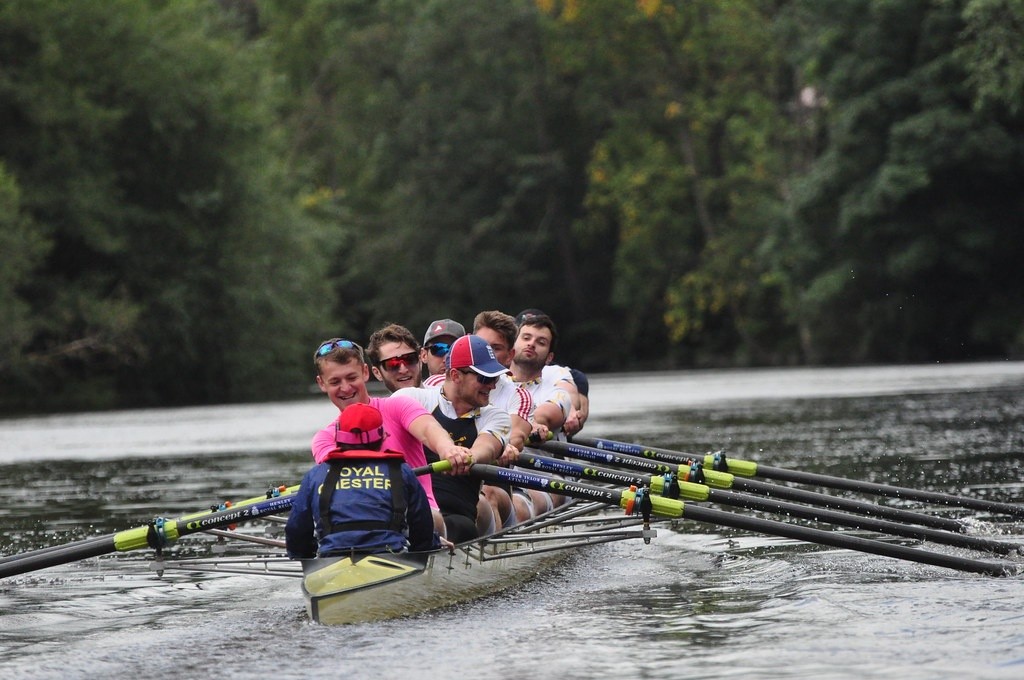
[282,310,591,561]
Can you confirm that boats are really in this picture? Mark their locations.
[285,440,645,628]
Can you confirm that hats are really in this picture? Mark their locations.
[446,333,513,377]
[340,403,384,433]
[424,319,465,345]
[514,309,546,326]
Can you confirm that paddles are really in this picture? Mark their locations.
[460,460,1022,577]
[1,481,304,577]
[512,430,1022,565]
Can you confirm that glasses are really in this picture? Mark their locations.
[457,369,500,385]
[316,340,359,358]
[374,351,420,371]
[424,342,450,357]
